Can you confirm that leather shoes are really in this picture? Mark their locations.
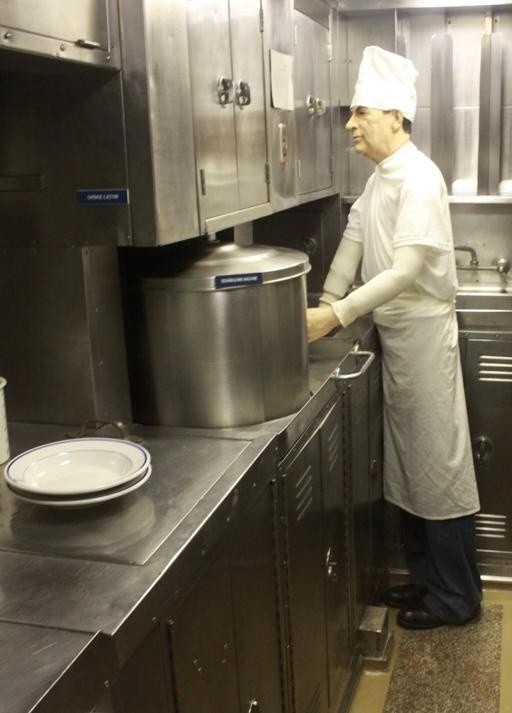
[397,603,446,629]
[384,584,424,607]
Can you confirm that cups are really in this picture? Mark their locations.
[1,376,11,463]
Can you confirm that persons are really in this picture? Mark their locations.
[305,106,483,631]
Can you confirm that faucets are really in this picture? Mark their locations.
[454,242,479,268]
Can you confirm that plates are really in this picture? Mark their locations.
[5,437,155,509]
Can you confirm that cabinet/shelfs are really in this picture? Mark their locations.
[0,0,122,72]
[457,333,512,589]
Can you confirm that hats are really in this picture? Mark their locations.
[351,46,420,121]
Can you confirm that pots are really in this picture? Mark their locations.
[125,243,313,429]
